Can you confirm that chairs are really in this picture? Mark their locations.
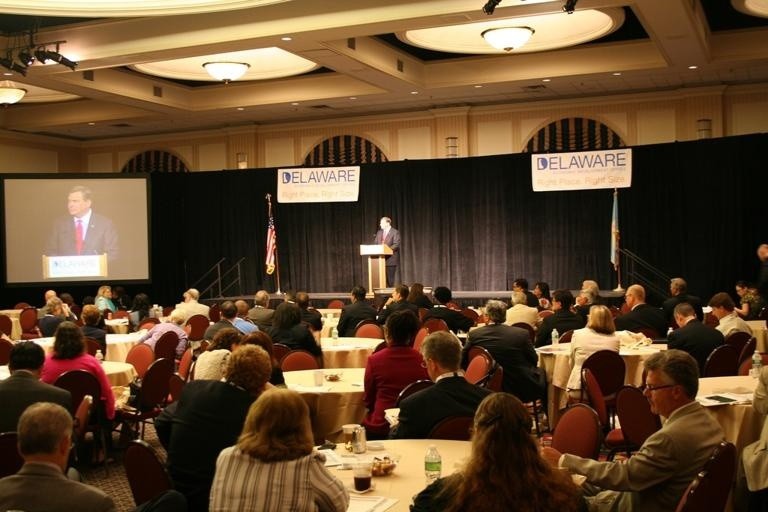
[396,378,438,409]
[355,319,385,338]
[3,429,29,477]
[2,314,16,340]
[138,317,161,331]
[109,347,195,442]
[271,343,292,367]
[548,348,663,464]
[423,306,767,337]
[53,369,113,481]
[681,440,741,512]
[184,313,209,342]
[21,309,42,339]
[208,304,220,325]
[125,342,156,380]
[112,310,133,333]
[699,328,758,378]
[464,344,542,437]
[280,350,317,374]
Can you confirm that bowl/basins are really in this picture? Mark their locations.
[362,455,401,475]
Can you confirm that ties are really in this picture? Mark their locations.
[75,219,83,256]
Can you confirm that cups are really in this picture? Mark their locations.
[340,457,357,469]
[540,440,552,447]
[353,462,372,491]
[106,312,114,321]
[315,372,324,386]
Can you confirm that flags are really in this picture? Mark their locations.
[608,196,622,269]
[264,217,279,276]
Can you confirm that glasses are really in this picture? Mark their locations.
[645,384,673,392]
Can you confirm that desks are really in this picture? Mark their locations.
[312,439,633,512]
[100,318,130,335]
[2,308,24,339]
[744,320,766,366]
[696,376,762,448]
[316,336,386,370]
[535,343,671,433]
[1,359,139,391]
[28,333,151,362]
[280,368,366,448]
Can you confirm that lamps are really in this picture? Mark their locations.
[201,62,251,84]
[481,26,534,52]
[0,87,28,110]
[0,17,78,77]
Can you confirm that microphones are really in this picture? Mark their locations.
[373,234,376,240]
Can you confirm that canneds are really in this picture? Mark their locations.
[352,426,367,454]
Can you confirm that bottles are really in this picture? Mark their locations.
[751,348,764,382]
[324,311,338,327]
[342,422,367,453]
[667,327,674,334]
[151,303,164,313]
[551,328,559,348]
[424,444,442,482]
[94,349,104,370]
[331,328,340,345]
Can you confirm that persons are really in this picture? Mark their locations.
[370,217,401,286]
[45,187,119,269]
[0,276,768,512]
